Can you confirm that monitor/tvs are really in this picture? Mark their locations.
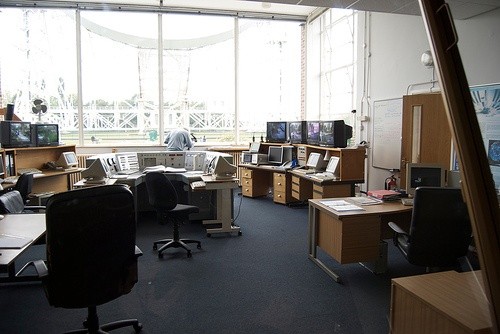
[53,152,78,171]
[0,121,31,148]
[267,122,287,143]
[319,120,352,148]
[32,124,59,147]
[288,121,306,144]
[81,157,110,183]
[305,121,319,145]
[211,155,238,174]
[306,153,327,172]
[406,163,446,198]
[325,156,340,177]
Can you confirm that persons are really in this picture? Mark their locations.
[163,127,193,151]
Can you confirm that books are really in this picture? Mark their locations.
[368,191,401,200]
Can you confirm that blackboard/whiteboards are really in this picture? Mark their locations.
[372,96,404,172]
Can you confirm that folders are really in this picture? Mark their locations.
[368,189,402,200]
[5,154,15,177]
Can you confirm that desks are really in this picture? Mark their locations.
[0,213,46,285]
[73,169,244,236]
[0,143,88,215]
[307,190,415,284]
[389,269,494,334]
[237,141,366,207]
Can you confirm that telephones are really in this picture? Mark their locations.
[282,161,294,169]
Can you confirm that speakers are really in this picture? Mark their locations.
[267,146,283,163]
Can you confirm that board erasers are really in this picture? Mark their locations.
[393,169,400,172]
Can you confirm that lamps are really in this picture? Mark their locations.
[421,50,433,67]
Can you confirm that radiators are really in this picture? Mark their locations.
[220,152,242,188]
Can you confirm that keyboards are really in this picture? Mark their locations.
[400,198,413,205]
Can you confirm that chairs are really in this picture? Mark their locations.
[33,184,144,334]
[387,187,474,275]
[144,172,202,260]
[0,171,47,214]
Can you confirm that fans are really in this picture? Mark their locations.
[27,97,50,125]
